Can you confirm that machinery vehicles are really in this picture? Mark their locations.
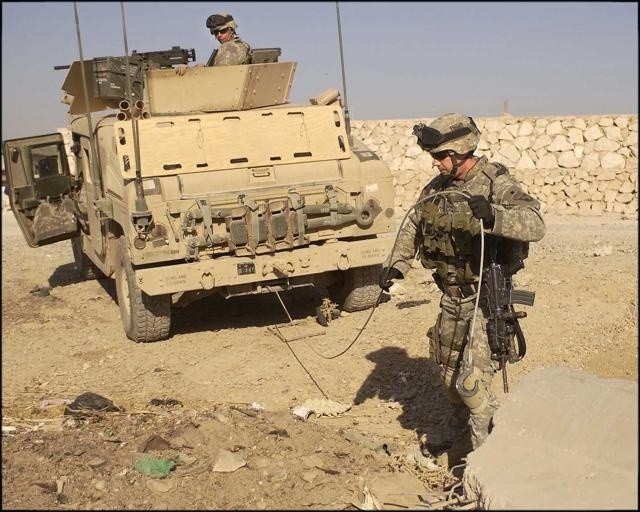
[4,2,395,341]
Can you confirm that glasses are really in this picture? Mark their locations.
[413,123,444,145]
[214,28,228,34]
[430,150,449,160]
[206,15,226,27]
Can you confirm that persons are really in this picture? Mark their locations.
[377,110,547,468]
[172,13,251,78]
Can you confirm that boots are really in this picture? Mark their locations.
[436,432,472,467]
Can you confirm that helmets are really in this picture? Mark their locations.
[210,11,236,35]
[417,112,481,154]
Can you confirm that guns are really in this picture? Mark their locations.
[476,234,535,395]
[54,46,195,67]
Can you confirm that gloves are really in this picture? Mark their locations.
[468,195,495,229]
[379,266,404,292]
[176,65,187,76]
